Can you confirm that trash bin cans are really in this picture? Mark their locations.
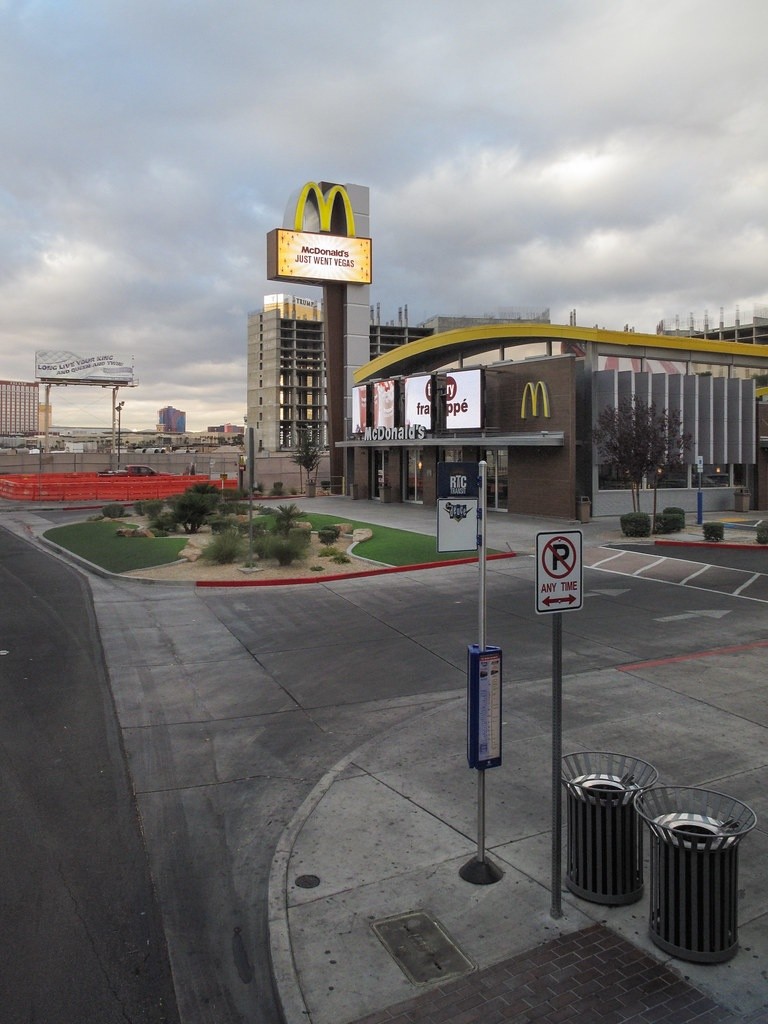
[304,479,317,498]
[733,488,752,512]
[349,484,358,500]
[561,751,660,907]
[377,482,392,503]
[576,496,592,523]
[634,785,759,967]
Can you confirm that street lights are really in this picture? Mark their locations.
[114,400,124,470]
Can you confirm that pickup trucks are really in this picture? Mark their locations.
[98,465,173,478]
